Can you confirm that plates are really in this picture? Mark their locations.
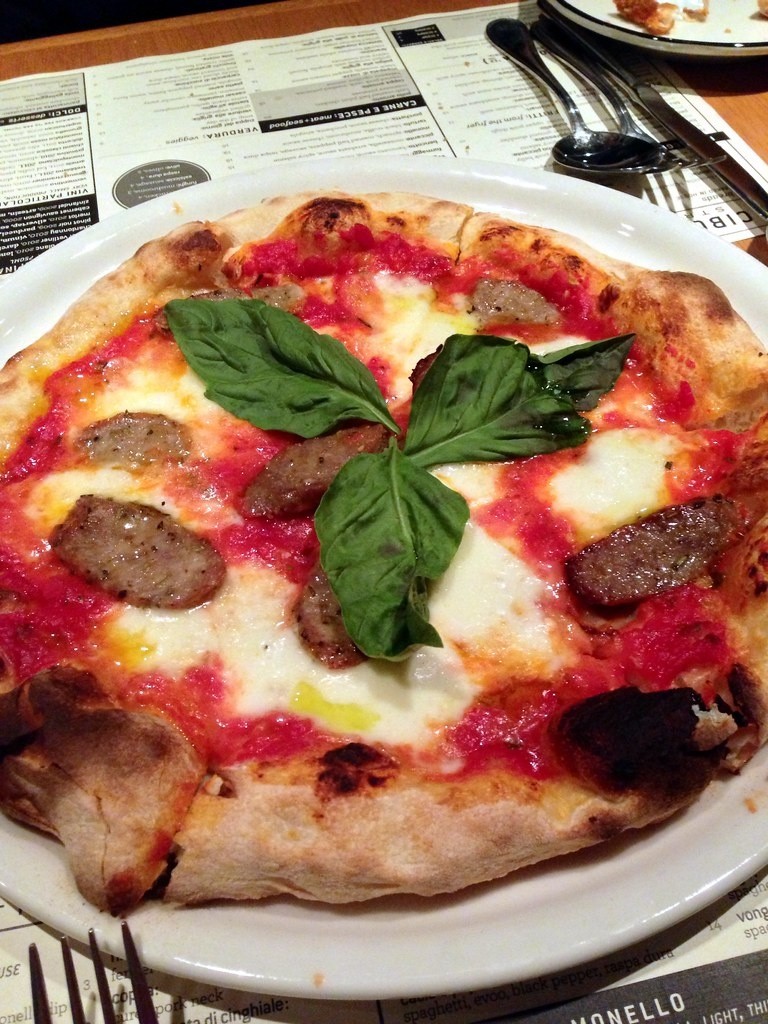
[1,166,768,1007]
[542,0,761,56]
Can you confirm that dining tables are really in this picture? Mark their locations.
[0,0,768,289]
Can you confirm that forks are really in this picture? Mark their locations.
[528,19,726,176]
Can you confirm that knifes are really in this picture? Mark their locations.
[537,1,768,226]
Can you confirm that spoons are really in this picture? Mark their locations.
[481,9,683,182]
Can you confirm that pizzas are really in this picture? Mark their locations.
[1,190,768,921]
